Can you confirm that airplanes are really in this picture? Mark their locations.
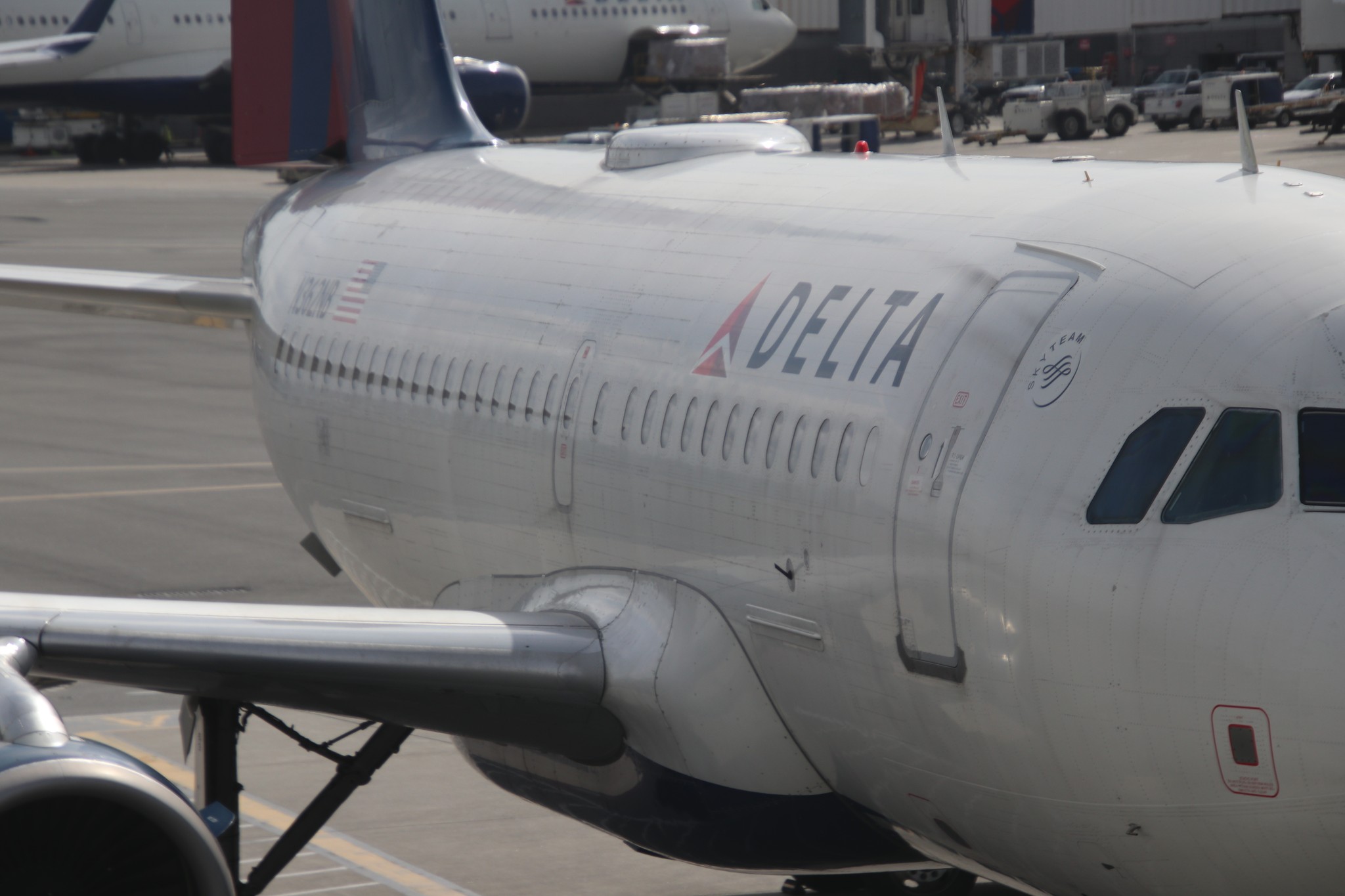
[0,3,1345,895]
[1,2,799,168]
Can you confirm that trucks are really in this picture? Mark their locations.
[1000,66,1345,143]
